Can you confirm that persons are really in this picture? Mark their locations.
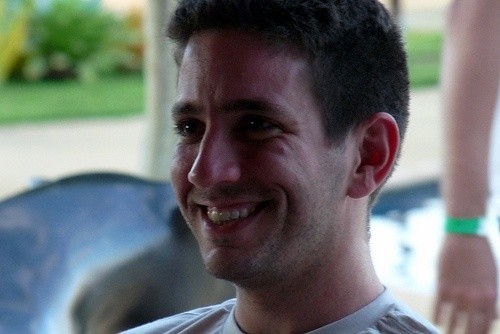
[114,0,445,334]
[429,0,500,334]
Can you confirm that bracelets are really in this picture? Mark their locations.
[442,216,491,237]
